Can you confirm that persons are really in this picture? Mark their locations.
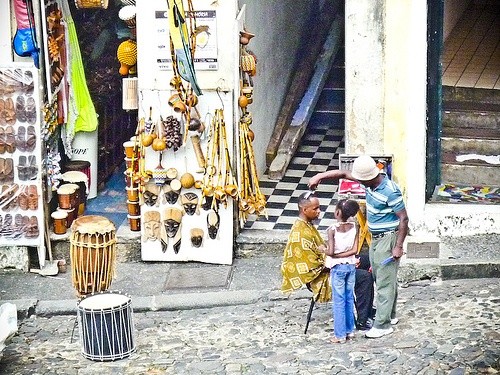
[319,198,360,343]
[280,191,377,330]
[307,155,409,339]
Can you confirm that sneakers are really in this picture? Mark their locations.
[331,332,354,343]
[365,327,394,338]
[390,318,399,325]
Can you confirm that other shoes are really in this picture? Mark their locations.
[356,320,374,330]
[369,305,377,314]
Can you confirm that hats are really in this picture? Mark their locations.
[351,156,379,181]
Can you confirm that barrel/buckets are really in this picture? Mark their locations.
[123,142,139,159]
[124,158,139,173]
[126,187,140,203]
[56,183,79,228]
[127,214,141,231]
[51,211,67,234]
[126,200,141,217]
[123,172,140,189]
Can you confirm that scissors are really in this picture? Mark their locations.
[304,185,319,199]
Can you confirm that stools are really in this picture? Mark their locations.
[304,282,358,334]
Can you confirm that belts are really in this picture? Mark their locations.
[371,233,384,238]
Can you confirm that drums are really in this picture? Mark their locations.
[70,215,116,297]
[70,292,137,362]
[118,5,136,37]
[123,136,141,231]
[51,160,91,235]
[74,0,109,10]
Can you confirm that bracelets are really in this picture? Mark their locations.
[323,248,327,252]
